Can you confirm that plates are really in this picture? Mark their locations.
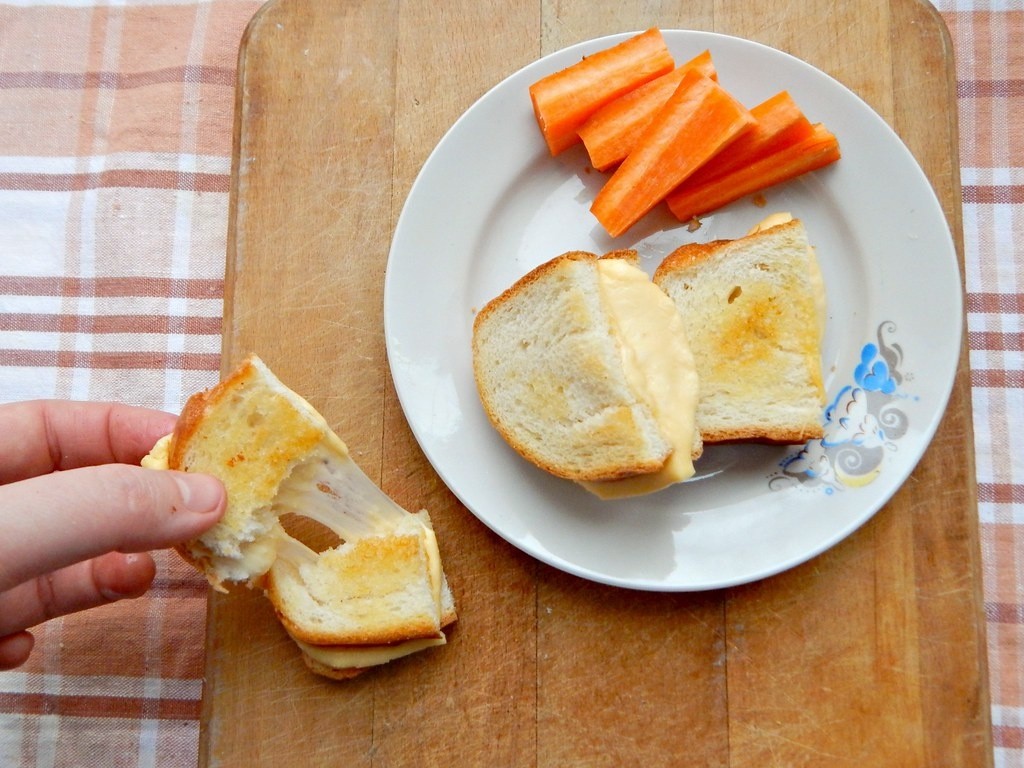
[385,30,958,593]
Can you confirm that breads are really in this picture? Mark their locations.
[136,211,829,683]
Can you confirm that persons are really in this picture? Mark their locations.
[0,399,228,674]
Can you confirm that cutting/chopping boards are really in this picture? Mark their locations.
[210,0,996,768]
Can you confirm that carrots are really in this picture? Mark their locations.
[529,26,843,237]
